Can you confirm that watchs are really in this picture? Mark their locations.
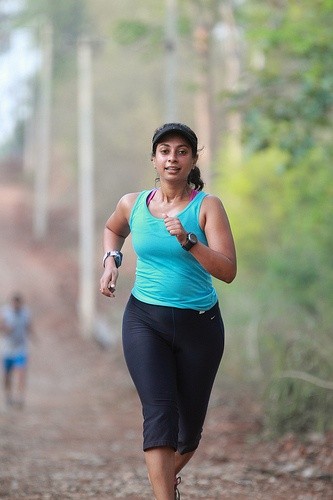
[103,250,123,268]
[182,232,199,252]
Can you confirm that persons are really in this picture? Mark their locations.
[0,292,38,409]
[99,122,238,500]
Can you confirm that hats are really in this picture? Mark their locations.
[152,125,197,156]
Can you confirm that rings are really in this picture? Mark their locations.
[99,289,101,291]
